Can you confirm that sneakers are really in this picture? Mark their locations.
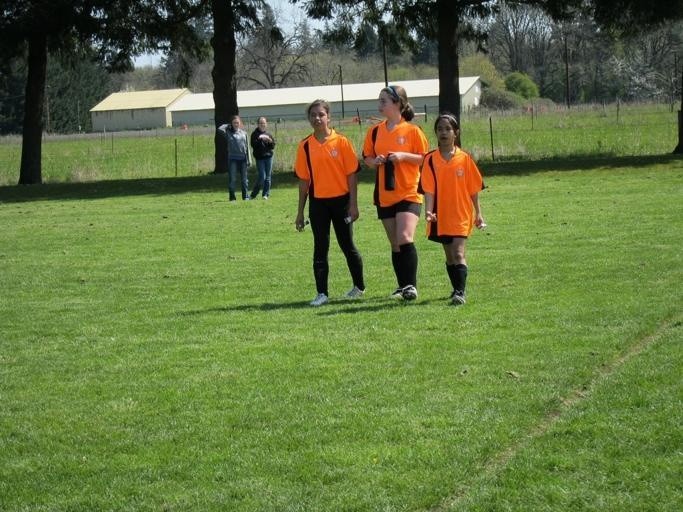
[388,287,405,301]
[309,293,329,306]
[347,284,366,297]
[402,284,418,300]
[452,289,466,305]
[230,194,268,202]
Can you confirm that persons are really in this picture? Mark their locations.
[416,113,487,305]
[295,98,366,306]
[362,85,431,301]
[216,115,252,202]
[249,117,276,199]
